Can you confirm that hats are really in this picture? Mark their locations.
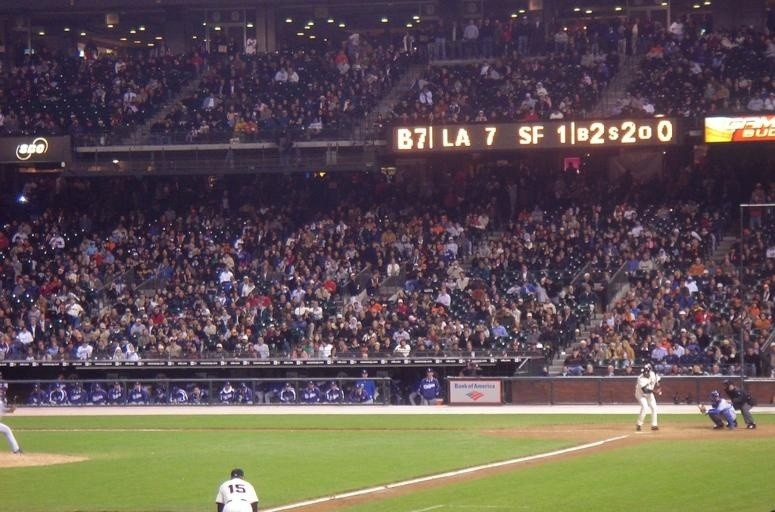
[230,468,244,478]
[0,366,440,394]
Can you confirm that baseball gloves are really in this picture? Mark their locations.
[699,403,707,415]
[8,405,16,413]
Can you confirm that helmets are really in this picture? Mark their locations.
[641,362,653,373]
[710,390,720,399]
[722,379,732,392]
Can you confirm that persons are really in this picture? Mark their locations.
[0,381,23,454]
[214,468,260,511]
[27,368,482,405]
[635,362,662,430]
[2,148,775,376]
[706,389,738,429]
[0,2,774,148]
[722,379,757,429]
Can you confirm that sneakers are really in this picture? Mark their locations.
[713,423,756,430]
[636,425,658,431]
